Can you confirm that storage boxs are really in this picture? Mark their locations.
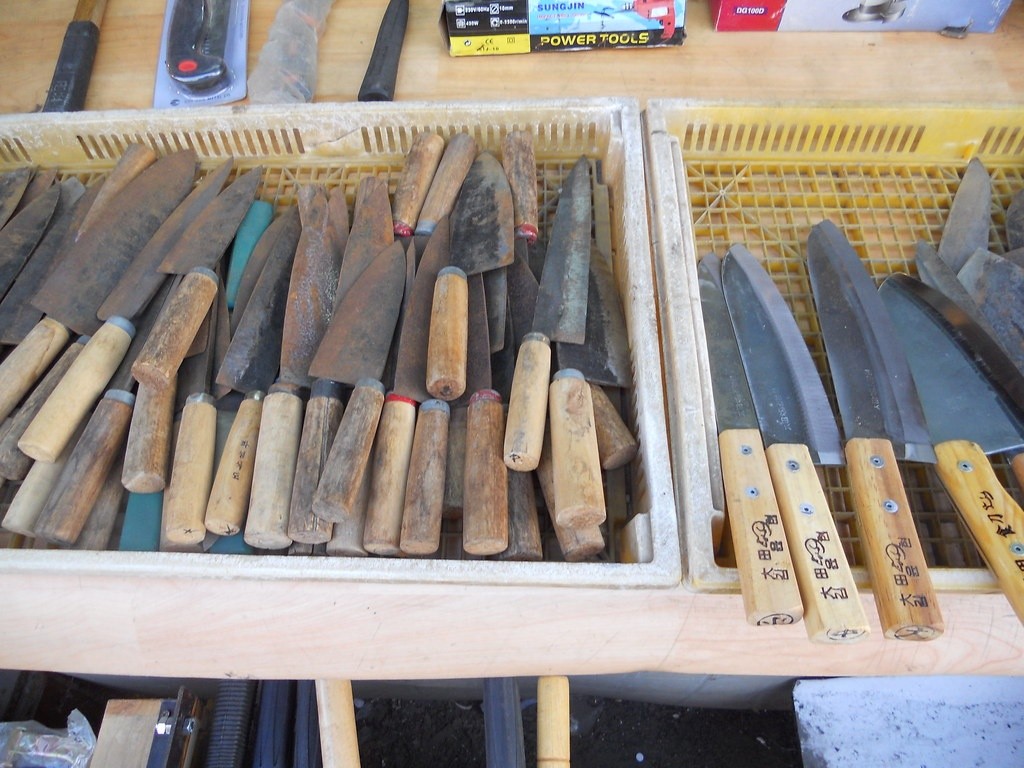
[0,95,1024,598]
[436,0,683,60]
[709,0,1016,32]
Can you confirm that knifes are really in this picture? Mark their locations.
[697,156,1024,642]
[1,132,636,562]
[42,0,106,112]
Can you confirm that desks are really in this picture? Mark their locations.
[1,0,1022,679]
